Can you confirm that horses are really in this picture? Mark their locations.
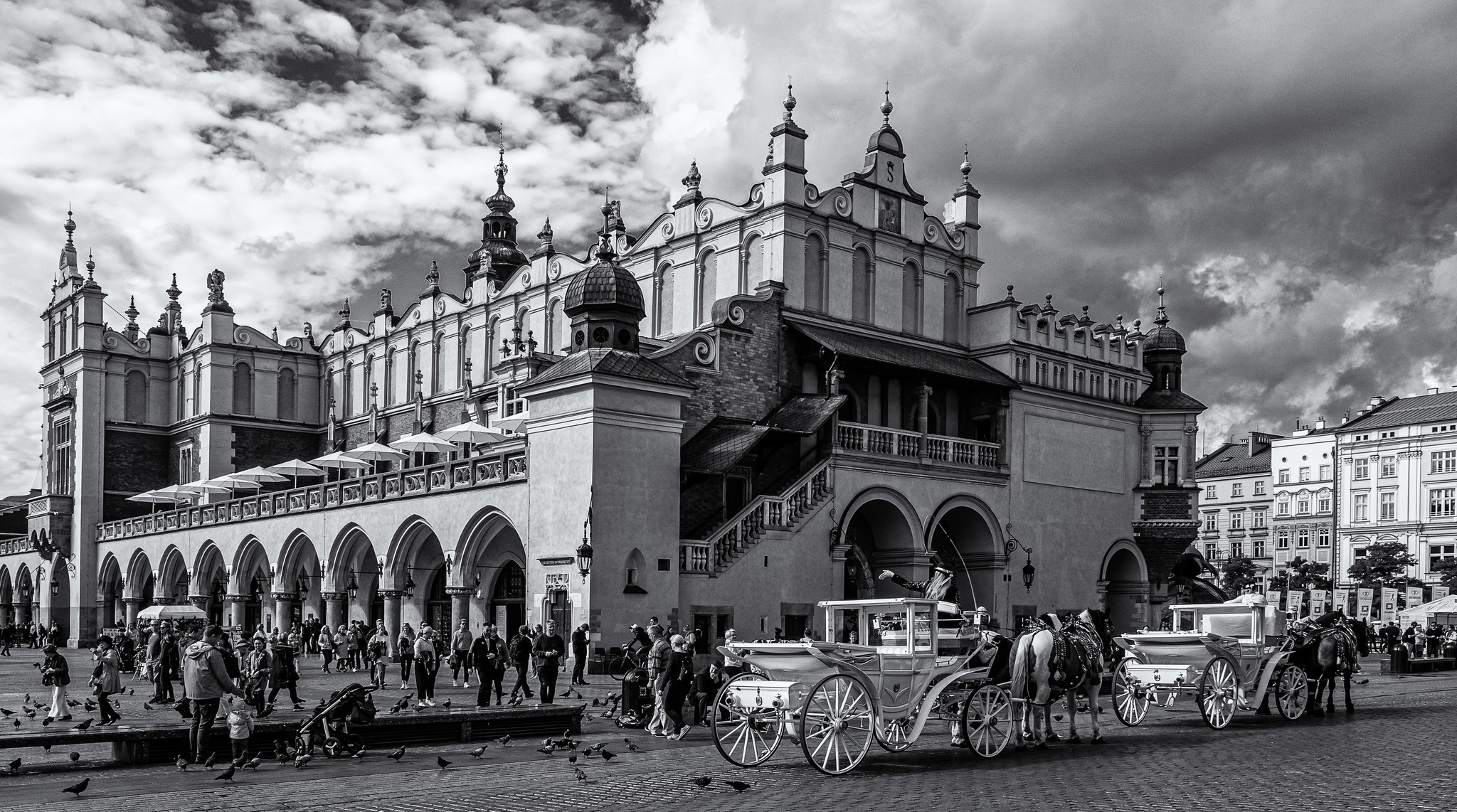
[1256,609,1347,718]
[1281,616,1376,719]
[946,612,1074,748]
[1008,607,1116,749]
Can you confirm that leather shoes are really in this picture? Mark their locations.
[721,718,736,726]
[699,720,710,727]
[44,713,121,726]
[572,680,590,686]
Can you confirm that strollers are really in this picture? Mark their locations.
[292,681,383,758]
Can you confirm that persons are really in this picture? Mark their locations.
[0,577,1457,767]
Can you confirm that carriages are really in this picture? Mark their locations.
[711,598,1106,778]
[1112,601,1370,729]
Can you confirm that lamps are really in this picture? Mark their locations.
[253,566,275,605]
[22,560,46,600]
[211,561,230,606]
[182,571,192,605]
[829,509,860,589]
[406,554,453,600]
[576,484,594,584]
[50,553,76,597]
[1004,523,1035,594]
[347,556,384,602]
[298,560,325,603]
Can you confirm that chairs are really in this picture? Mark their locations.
[591,646,645,675]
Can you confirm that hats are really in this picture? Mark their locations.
[40,643,57,652]
[628,624,638,629]
[234,638,250,650]
[233,697,245,710]
[96,635,113,647]
[712,660,723,668]
[934,564,954,586]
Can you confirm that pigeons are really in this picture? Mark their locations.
[1356,678,1369,686]
[0,685,754,792]
[1050,696,1105,727]
[1395,674,1405,681]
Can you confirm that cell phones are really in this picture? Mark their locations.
[86,643,94,652]
[550,650,555,655]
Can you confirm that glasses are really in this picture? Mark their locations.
[483,628,493,632]
[648,632,653,637]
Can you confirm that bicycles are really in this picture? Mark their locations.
[606,643,654,681]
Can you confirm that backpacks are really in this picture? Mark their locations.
[672,652,693,685]
[508,634,529,660]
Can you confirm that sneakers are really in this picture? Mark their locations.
[265,703,306,712]
[321,662,410,690]
[148,695,176,705]
[645,726,658,735]
[667,733,680,741]
[418,680,535,708]
[678,725,691,740]
[655,732,673,738]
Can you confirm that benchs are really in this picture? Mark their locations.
[878,617,980,649]
